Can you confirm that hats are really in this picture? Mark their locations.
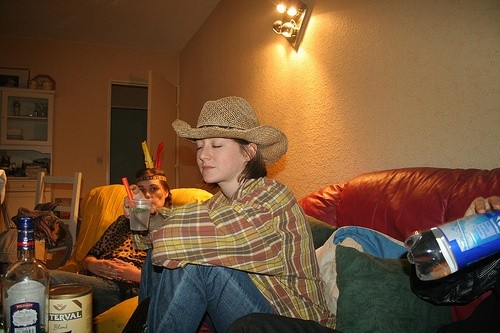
[172,96,288,166]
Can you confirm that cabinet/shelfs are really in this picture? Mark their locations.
[0,87,56,234]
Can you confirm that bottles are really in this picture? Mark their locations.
[2,217,50,333]
[14,100,20,116]
[403,209,500,281]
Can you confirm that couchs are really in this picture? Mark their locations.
[197,167,500,333]
[50,184,214,333]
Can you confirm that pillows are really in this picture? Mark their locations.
[306,214,337,249]
[335,244,452,333]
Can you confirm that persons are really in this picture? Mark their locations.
[410,195,500,333]
[47,169,175,317]
[122,96,330,333]
[226,313,347,333]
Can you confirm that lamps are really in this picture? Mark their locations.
[273,0,307,47]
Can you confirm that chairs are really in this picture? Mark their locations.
[35,171,82,269]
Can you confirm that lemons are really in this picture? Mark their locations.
[133,208,150,214]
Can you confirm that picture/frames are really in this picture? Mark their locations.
[0,67,30,89]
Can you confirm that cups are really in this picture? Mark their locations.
[128,199,153,230]
[28,80,37,89]
[42,81,51,90]
